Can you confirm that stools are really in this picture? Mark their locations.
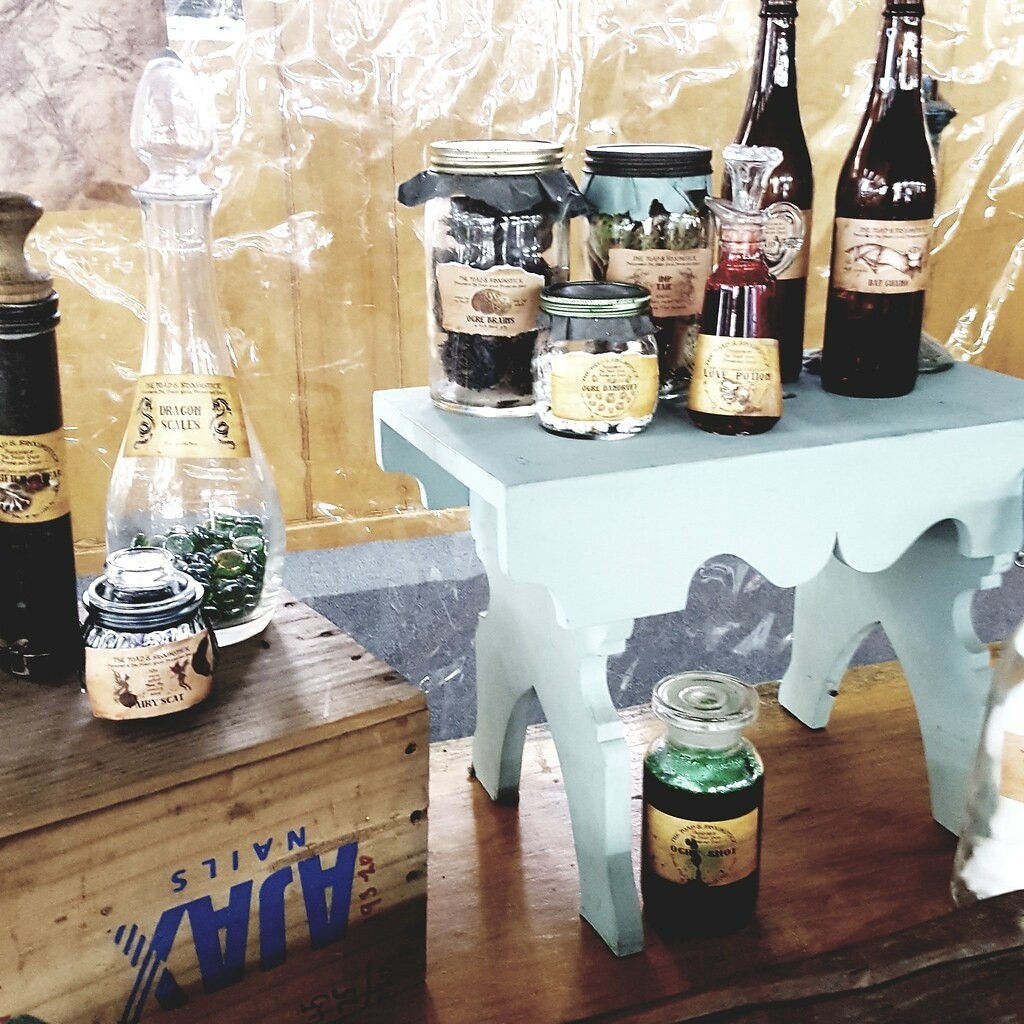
[370,351,1024,958]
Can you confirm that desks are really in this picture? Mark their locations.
[426,631,1024,1023]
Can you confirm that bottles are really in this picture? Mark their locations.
[0,192,84,681]
[76,548,216,739]
[720,0,815,383]
[687,144,785,436]
[640,671,766,933]
[822,0,937,399]
[105,58,284,646]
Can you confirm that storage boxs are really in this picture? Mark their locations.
[0,581,436,1024]
